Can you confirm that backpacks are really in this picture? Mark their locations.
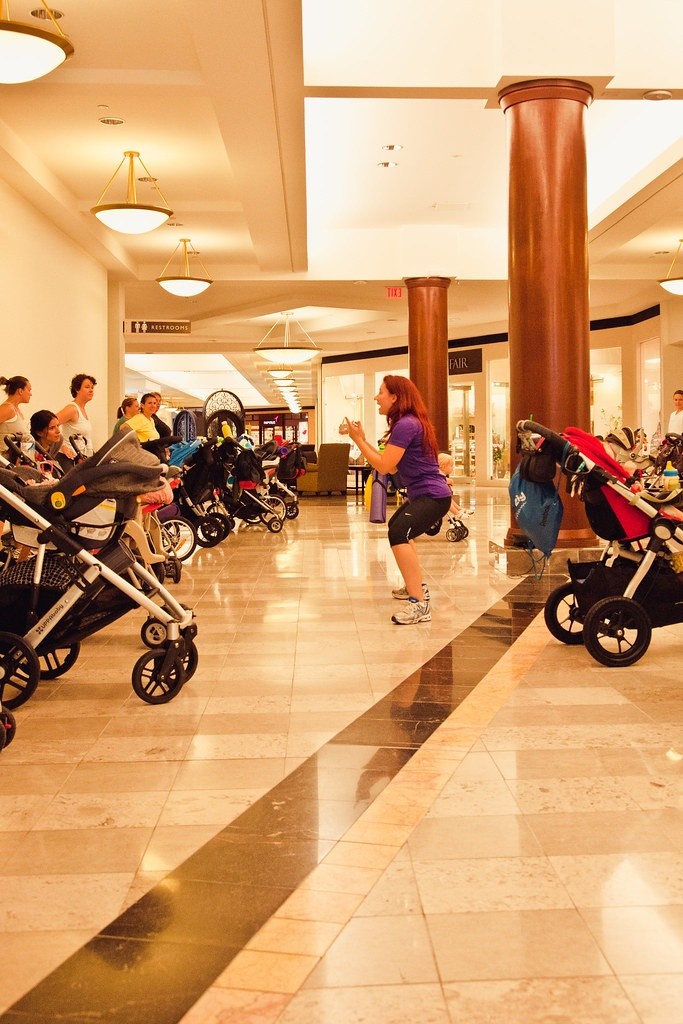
[507,436,571,582]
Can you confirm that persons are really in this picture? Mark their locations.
[0,372,98,561]
[344,375,451,624]
[438,452,474,519]
[668,390,683,488]
[114,391,172,462]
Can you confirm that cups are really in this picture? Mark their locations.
[529,433,541,447]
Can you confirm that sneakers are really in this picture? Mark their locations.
[391,583,430,601]
[391,596,432,625]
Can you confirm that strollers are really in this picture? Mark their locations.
[395,486,469,543]
[0,432,306,753]
[516,420,683,667]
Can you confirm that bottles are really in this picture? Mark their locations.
[662,461,680,491]
[221,421,232,438]
[379,442,386,455]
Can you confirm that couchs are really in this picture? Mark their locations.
[295,442,351,497]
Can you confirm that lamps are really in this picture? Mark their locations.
[155,237,214,298]
[273,377,304,414]
[166,401,179,412]
[266,366,293,378]
[253,310,321,365]
[659,237,683,296]
[90,150,176,235]
[158,397,169,410]
[0,0,76,84]
[175,405,186,414]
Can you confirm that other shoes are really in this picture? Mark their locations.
[455,509,474,520]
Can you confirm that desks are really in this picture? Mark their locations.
[348,464,370,496]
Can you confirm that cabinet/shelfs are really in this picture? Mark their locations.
[452,439,475,477]
